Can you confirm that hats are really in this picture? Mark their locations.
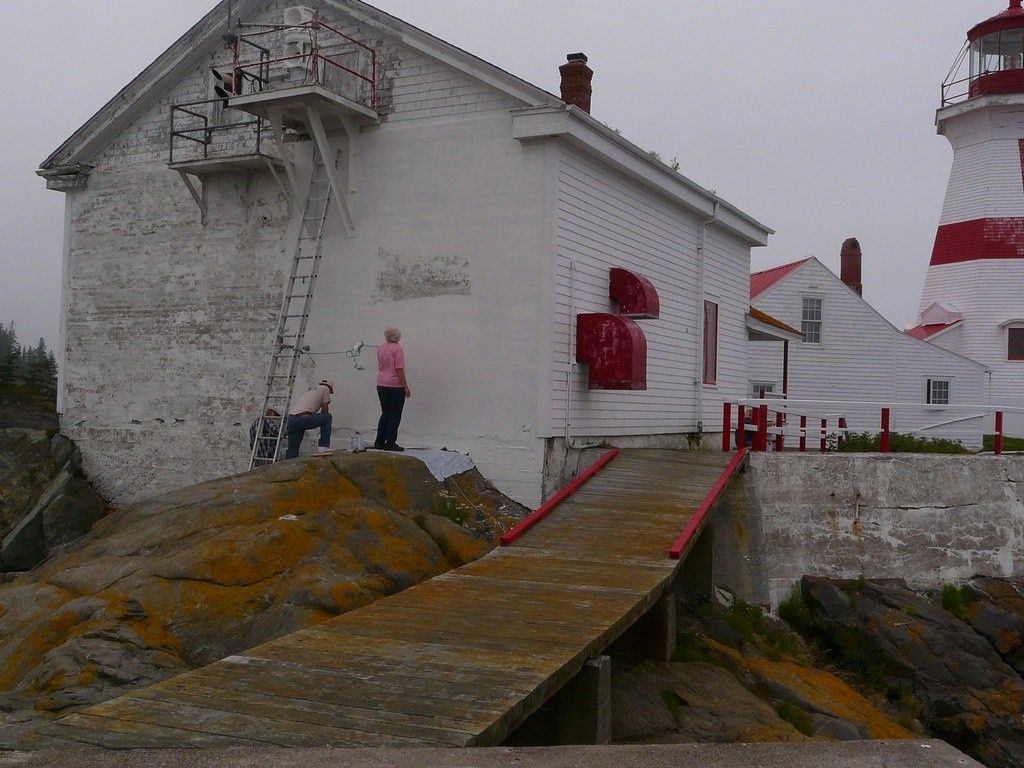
[319,378,333,394]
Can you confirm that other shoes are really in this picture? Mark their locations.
[384,443,404,451]
[376,442,385,449]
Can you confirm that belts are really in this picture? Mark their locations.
[289,411,312,417]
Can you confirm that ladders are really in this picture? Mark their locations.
[247,148,342,470]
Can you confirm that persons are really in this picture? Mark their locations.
[249,409,283,469]
[285,380,334,460]
[374,327,411,452]
